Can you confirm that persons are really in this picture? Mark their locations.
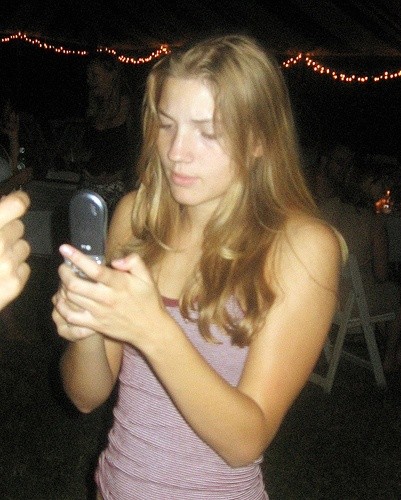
[299,123,401,379]
[51,33,351,500]
[1,52,136,193]
[0,189,32,312]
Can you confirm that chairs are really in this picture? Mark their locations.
[307,254,396,395]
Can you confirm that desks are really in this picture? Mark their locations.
[20,181,78,261]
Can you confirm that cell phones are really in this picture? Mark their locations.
[63,190,110,283]
